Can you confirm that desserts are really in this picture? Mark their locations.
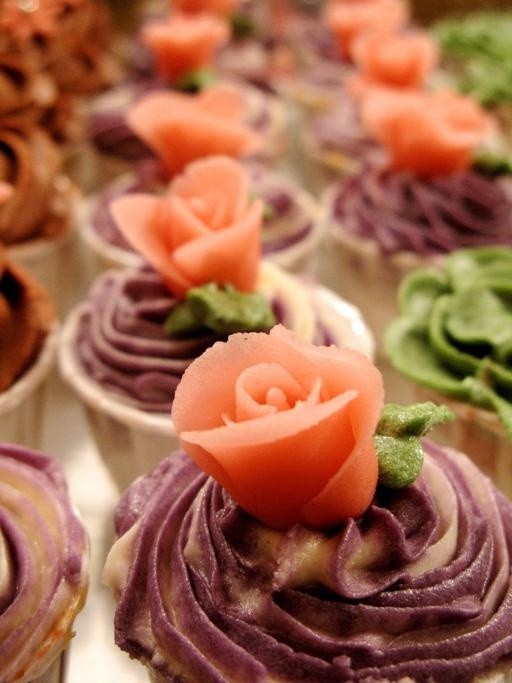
[0,1,512,683]
